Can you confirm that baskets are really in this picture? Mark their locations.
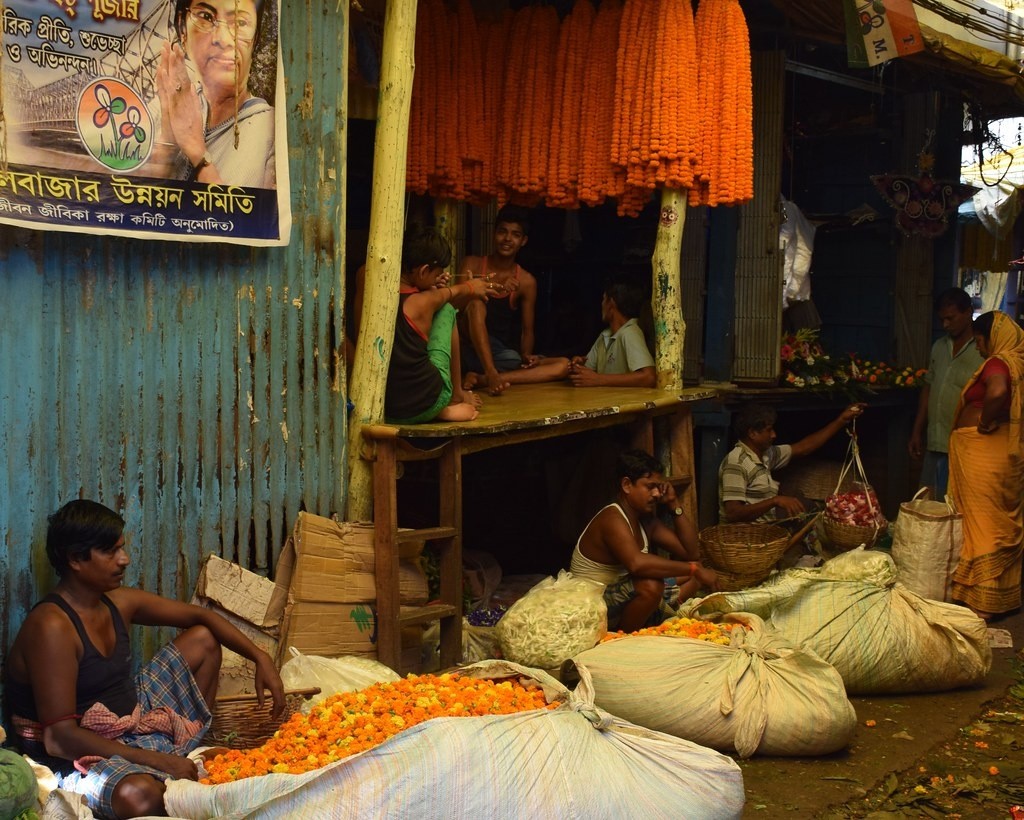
[198,693,304,751]
[698,522,793,593]
[772,454,857,501]
[821,510,875,548]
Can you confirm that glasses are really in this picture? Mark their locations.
[186,6,259,43]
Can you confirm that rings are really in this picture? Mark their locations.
[485,275,490,281]
[176,86,182,92]
[489,282,493,288]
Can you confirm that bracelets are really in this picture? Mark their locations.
[688,562,697,576]
[465,281,474,296]
[157,141,176,147]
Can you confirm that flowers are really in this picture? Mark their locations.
[822,486,885,528]
[600,613,751,647]
[782,328,929,399]
[194,670,561,785]
[468,605,509,627]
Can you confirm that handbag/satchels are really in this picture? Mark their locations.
[890,487,964,602]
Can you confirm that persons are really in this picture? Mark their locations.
[568,451,725,634]
[718,403,868,525]
[570,283,656,524]
[346,232,504,421]
[907,285,986,503]
[946,309,1024,621]
[145,0,277,190]
[454,204,571,395]
[0,499,285,820]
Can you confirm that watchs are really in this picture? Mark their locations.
[193,152,211,178]
[670,506,683,516]
